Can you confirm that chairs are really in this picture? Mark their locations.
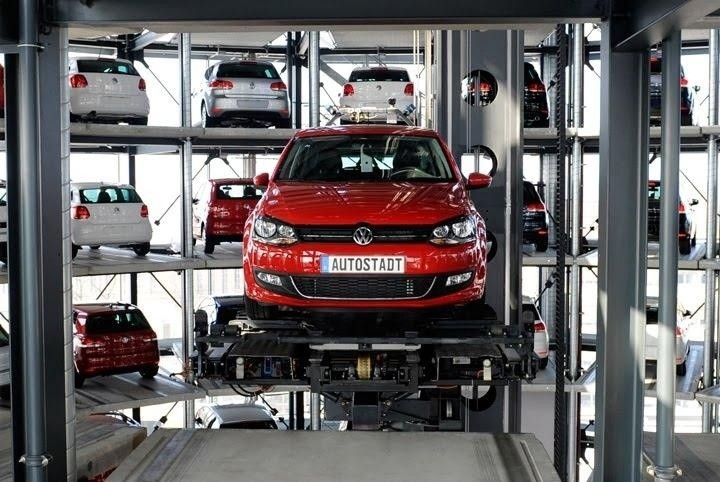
[313,148,344,181]
[243,187,256,197]
[387,147,423,181]
[98,192,111,202]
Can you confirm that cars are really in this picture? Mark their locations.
[245,125,493,319]
[0,64,11,398]
[195,403,280,430]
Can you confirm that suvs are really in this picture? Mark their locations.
[70,182,152,257]
[522,180,549,252]
[645,296,692,376]
[462,62,549,127]
[197,296,279,337]
[649,54,701,125]
[192,177,267,253]
[521,296,549,369]
[73,303,159,388]
[200,59,292,129]
[68,56,149,125]
[649,180,699,255]
[337,67,417,126]
